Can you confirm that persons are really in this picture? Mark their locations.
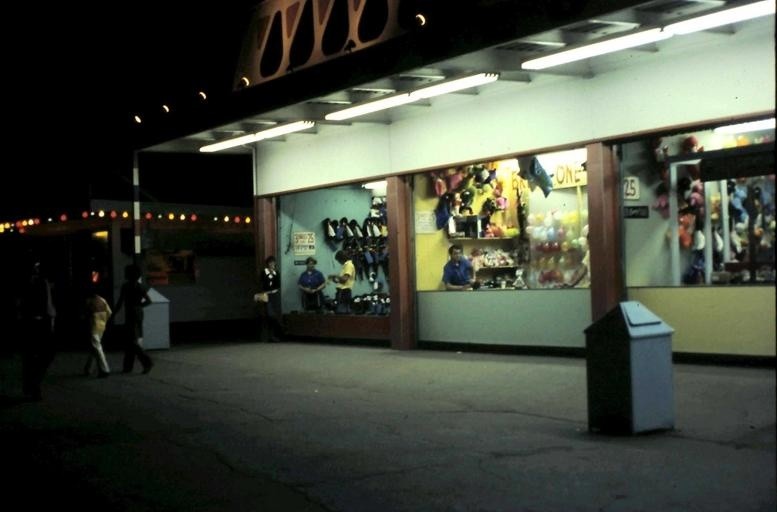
[74,282,114,376]
[296,256,327,311]
[561,245,587,288]
[441,244,477,290]
[253,254,281,342]
[111,264,154,374]
[18,259,62,396]
[328,250,356,305]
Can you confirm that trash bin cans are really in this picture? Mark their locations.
[585,301,674,434]
[141,284,172,352]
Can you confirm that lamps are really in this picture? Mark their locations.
[519,1,776,71]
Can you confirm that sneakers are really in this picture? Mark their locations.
[141,364,155,374]
[97,370,110,378]
[122,366,132,373]
[78,370,90,375]
[24,387,42,401]
